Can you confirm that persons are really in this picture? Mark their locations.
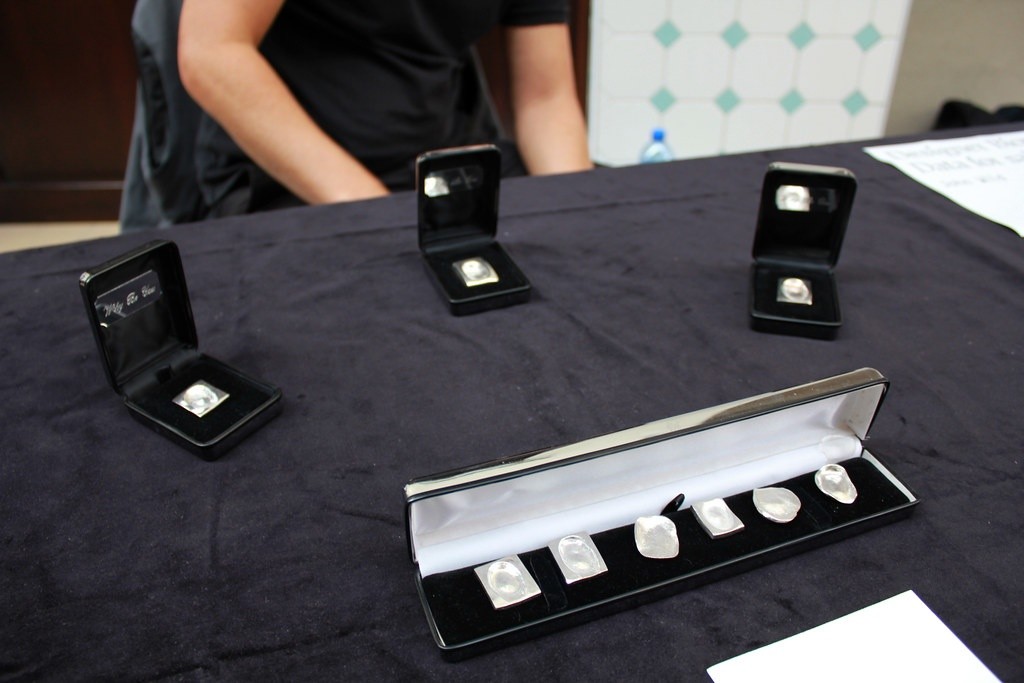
[119,0,615,235]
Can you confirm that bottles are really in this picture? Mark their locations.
[640,129,674,164]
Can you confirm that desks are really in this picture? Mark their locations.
[0,123,1024,683]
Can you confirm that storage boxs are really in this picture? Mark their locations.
[77,141,919,660]
